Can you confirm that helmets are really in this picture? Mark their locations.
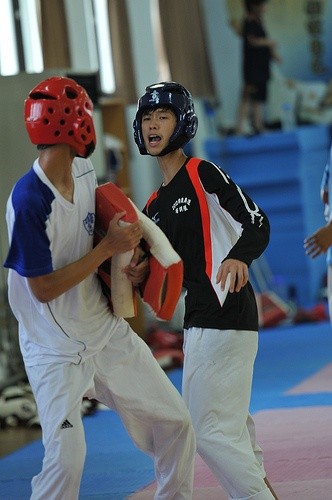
[133,82,199,155]
[25,76,97,159]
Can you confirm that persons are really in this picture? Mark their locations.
[3,76,197,500]
[132,81,332,500]
[230,0,281,138]
